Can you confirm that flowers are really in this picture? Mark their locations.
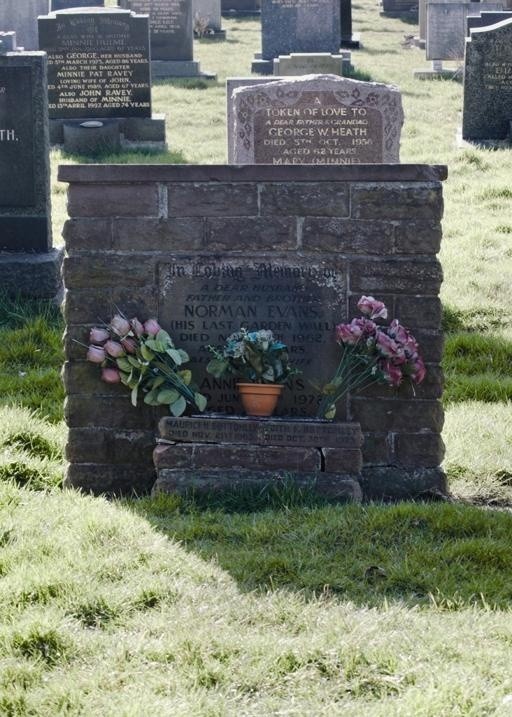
[207,326,302,385]
[319,295,426,421]
[71,301,208,415]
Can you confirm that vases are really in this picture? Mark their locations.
[236,383,283,416]
